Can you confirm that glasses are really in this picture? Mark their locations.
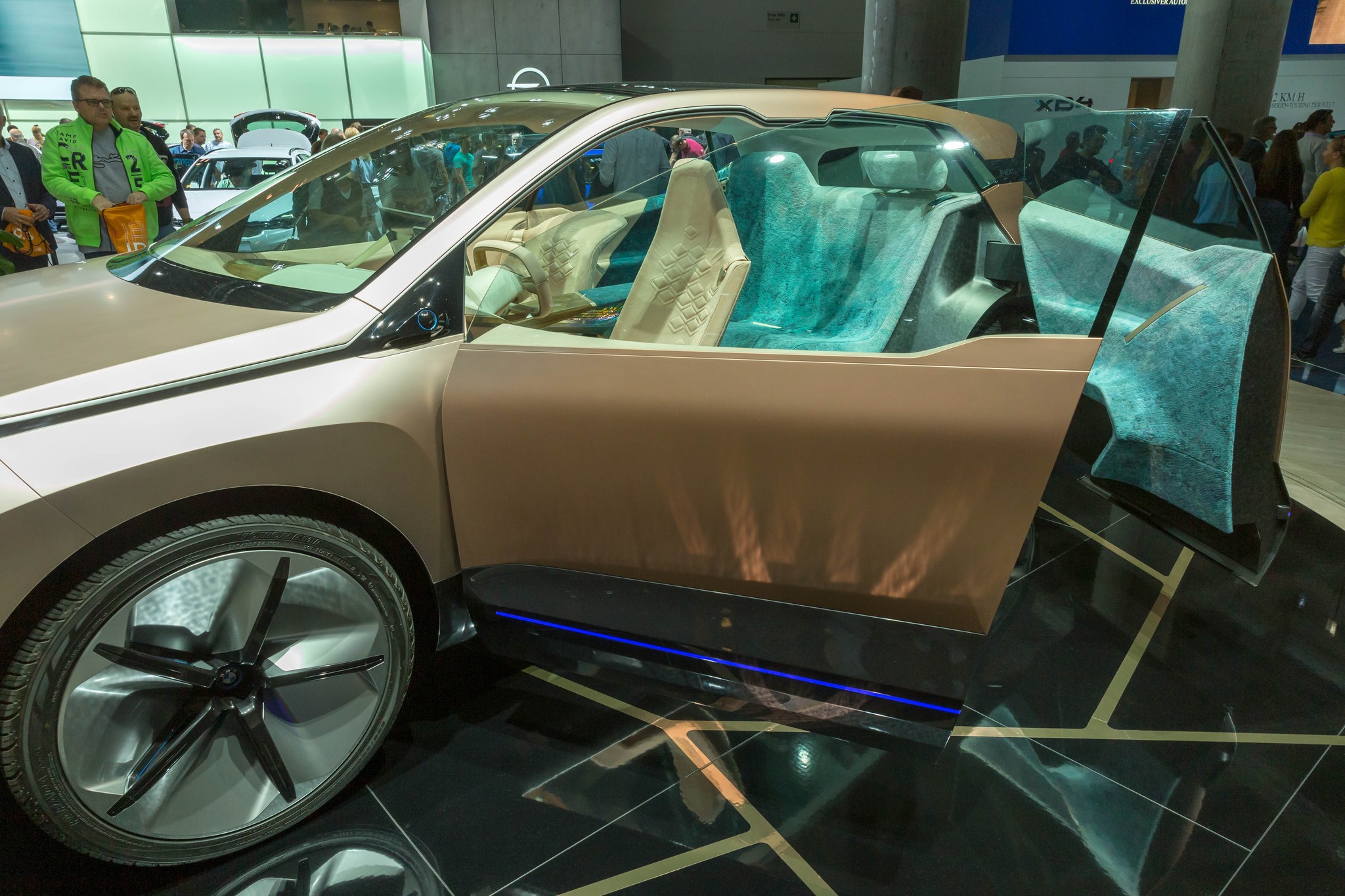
[77,98,113,108]
[1267,126,1277,131]
[110,87,137,97]
[677,130,682,134]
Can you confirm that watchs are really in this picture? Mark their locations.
[182,217,194,223]
[48,208,52,214]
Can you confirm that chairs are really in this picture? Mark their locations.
[607,158,753,347]
[500,210,627,299]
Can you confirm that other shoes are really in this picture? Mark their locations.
[1291,352,1316,361]
[1333,335,1345,353]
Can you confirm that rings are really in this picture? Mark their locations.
[19,220,21,223]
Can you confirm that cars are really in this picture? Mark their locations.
[164,107,323,252]
[0,82,1292,869]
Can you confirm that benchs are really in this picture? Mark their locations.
[718,151,980,354]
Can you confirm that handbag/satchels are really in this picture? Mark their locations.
[98,201,148,254]
[2,209,59,266]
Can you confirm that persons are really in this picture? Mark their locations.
[1289,129,1345,359]
[41,77,193,260]
[169,122,741,248]
[316,21,378,36]
[863,86,926,184]
[1022,110,1336,287]
[0,100,59,276]
